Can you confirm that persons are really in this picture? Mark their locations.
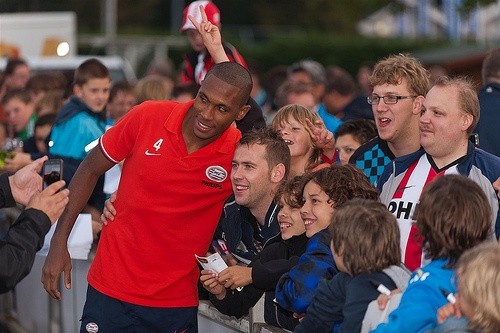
[368,176,492,332]
[376,75,500,271]
[269,103,341,180]
[180,2,266,136]
[0,1,499,228]
[100,128,292,317]
[435,241,500,332]
[276,165,376,330]
[47,58,110,214]
[332,118,378,176]
[0,156,69,292]
[40,61,254,332]
[201,175,310,331]
[299,199,412,332]
[349,55,436,199]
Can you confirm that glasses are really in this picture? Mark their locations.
[366,95,417,104]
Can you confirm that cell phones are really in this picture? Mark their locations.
[42,159,63,193]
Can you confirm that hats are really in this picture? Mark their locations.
[180,0,221,33]
[287,60,326,83]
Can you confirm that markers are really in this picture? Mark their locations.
[437,284,456,306]
[366,275,391,296]
